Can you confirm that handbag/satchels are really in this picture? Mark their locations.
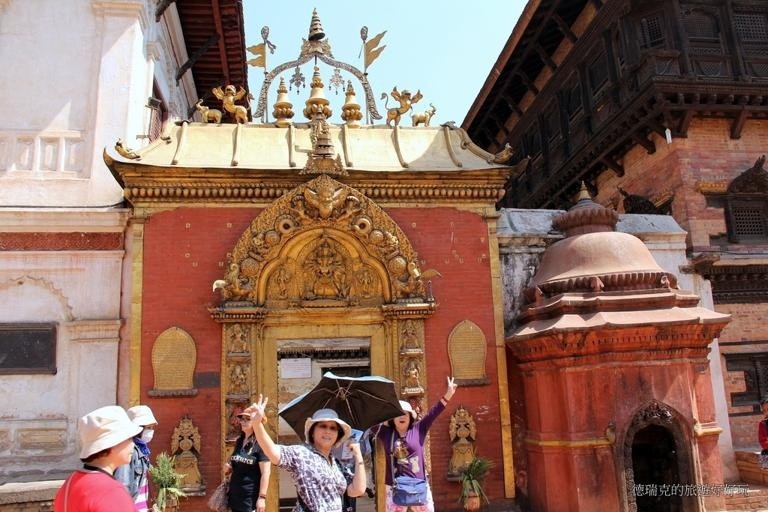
[206,479,231,512]
[390,475,429,506]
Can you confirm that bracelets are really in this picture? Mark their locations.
[259,495,266,499]
[442,396,450,403]
[356,461,364,465]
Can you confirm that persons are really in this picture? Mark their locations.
[371,376,457,512]
[247,394,367,512]
[53,405,143,512]
[112,405,158,512]
[222,404,270,512]
[758,394,768,469]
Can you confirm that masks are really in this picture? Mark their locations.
[138,427,157,444]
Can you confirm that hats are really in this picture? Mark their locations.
[76,404,158,461]
[301,408,352,448]
[237,405,270,425]
[398,399,418,419]
